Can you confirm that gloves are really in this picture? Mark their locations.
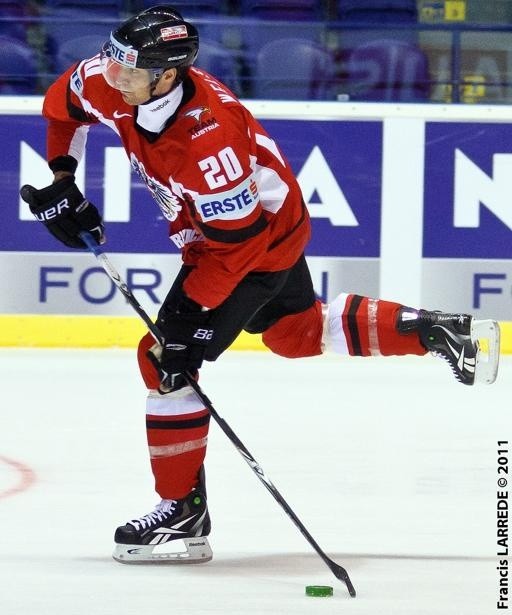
[19,174,108,249]
[146,294,218,396]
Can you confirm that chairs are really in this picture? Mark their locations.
[2,0,432,101]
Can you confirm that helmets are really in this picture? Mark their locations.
[100,5,199,93]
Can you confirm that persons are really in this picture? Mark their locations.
[28,4,477,546]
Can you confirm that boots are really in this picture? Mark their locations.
[397,305,479,385]
[115,465,211,545]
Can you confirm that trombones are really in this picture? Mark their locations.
[20,184,356,597]
